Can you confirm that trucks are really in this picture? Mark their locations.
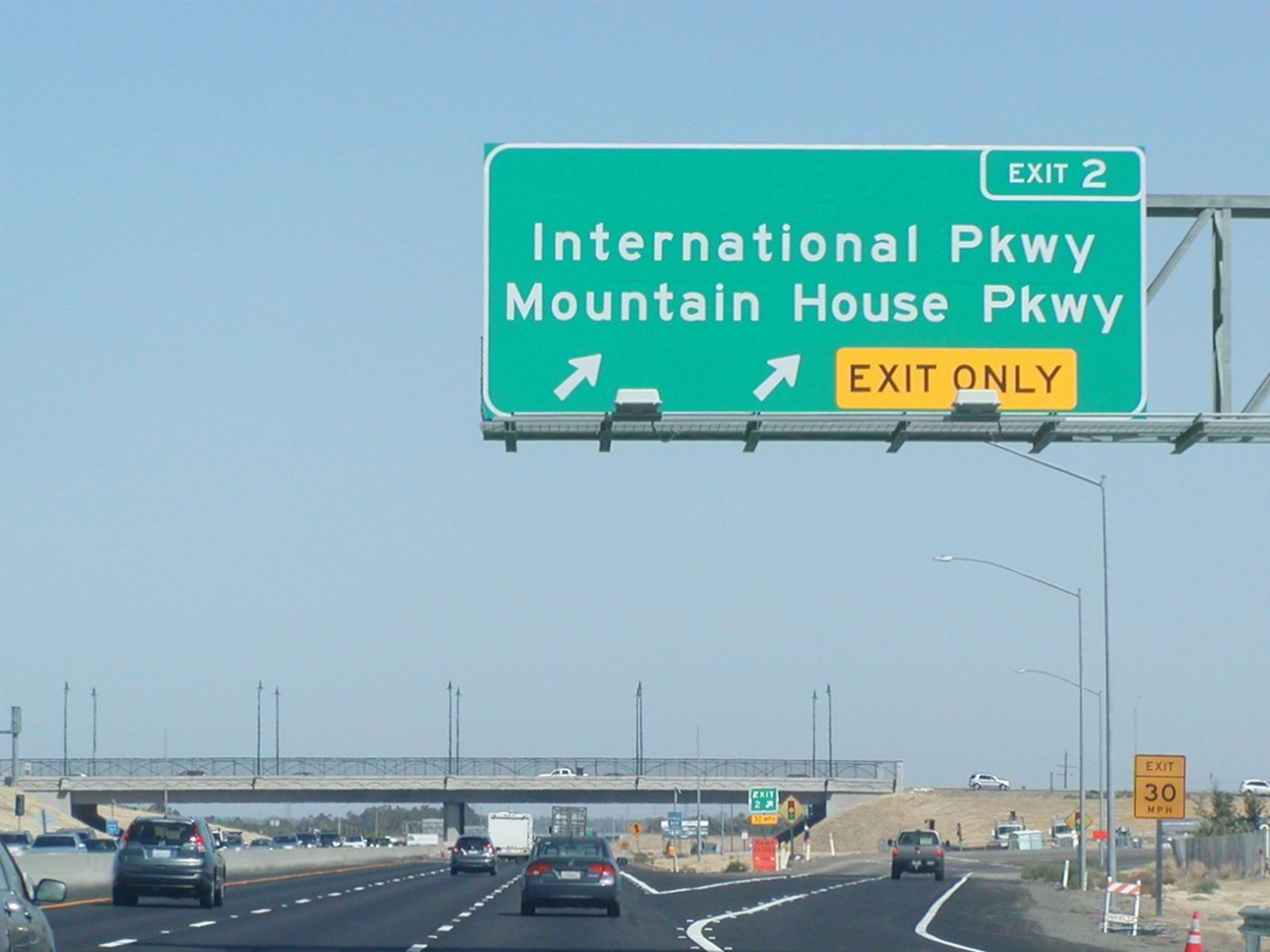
[993,817,1023,845]
[486,812,538,863]
[550,805,588,840]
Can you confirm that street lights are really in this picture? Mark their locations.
[812,689,818,777]
[255,679,264,775]
[446,680,453,774]
[89,687,98,776]
[274,686,281,775]
[62,681,70,778]
[455,688,462,776]
[1016,668,1107,872]
[826,683,834,776]
[634,682,645,775]
[932,553,1092,892]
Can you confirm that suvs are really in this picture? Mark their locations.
[969,774,1010,791]
[1238,780,1270,796]
[110,815,228,910]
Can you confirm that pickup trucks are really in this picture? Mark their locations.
[889,830,953,882]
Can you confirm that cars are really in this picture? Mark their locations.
[447,835,502,876]
[538,766,590,777]
[211,828,441,856]
[1051,824,1078,847]
[177,770,208,777]
[517,837,627,919]
[0,842,70,952]
[1,827,119,857]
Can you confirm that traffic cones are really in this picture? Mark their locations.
[1184,910,1202,952]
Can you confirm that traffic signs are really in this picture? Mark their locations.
[483,141,1144,428]
[1135,777,1184,819]
